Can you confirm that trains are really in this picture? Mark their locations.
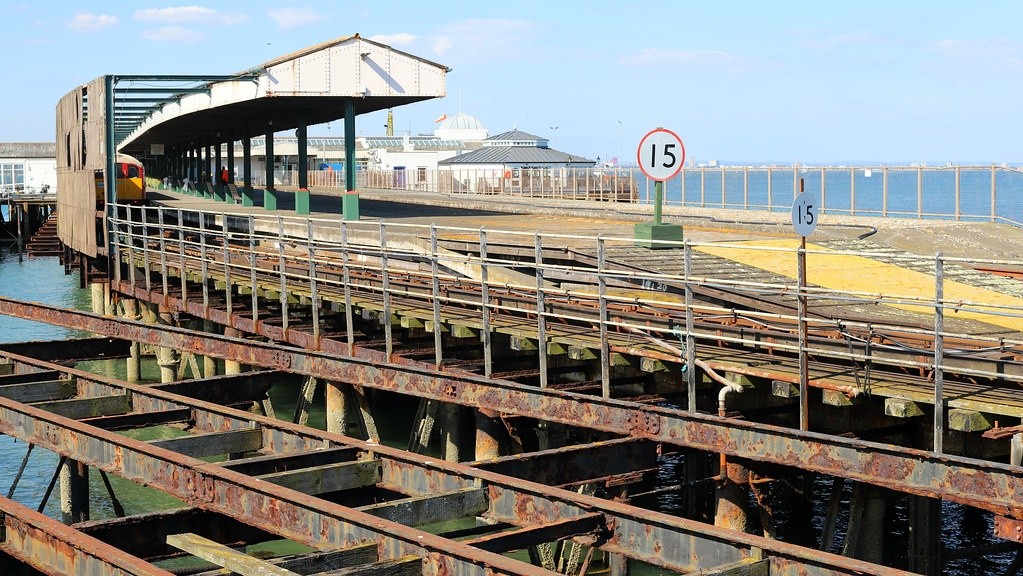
[96,152,147,220]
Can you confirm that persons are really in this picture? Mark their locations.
[221,166,228,185]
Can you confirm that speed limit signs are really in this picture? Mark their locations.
[637,128,686,182]
